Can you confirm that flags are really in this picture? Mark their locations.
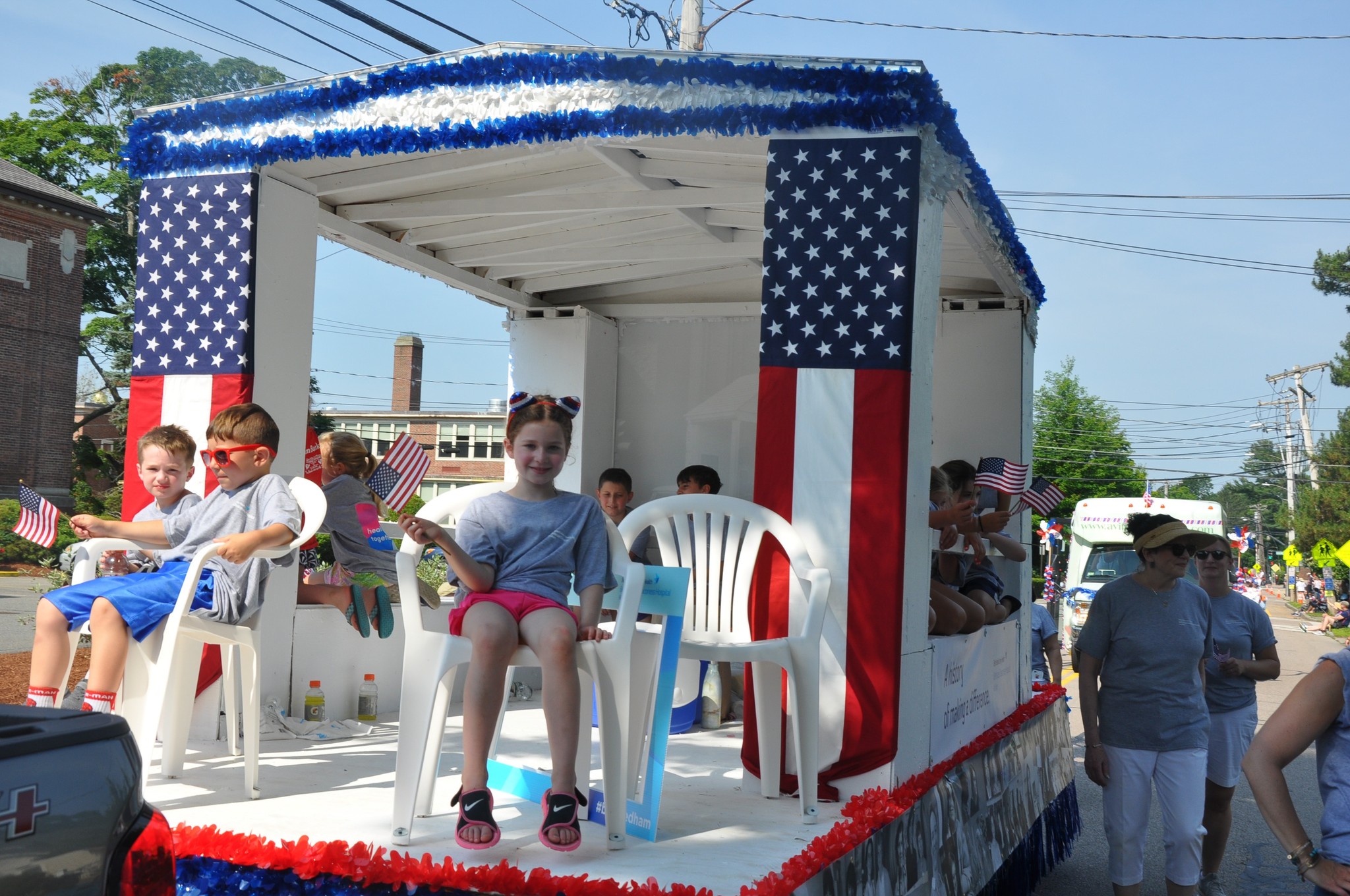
[364,431,431,513]
[1019,475,1066,518]
[11,483,60,549]
[1143,482,1153,504]
[974,457,1029,495]
[1012,500,1031,515]
[1212,645,1230,662]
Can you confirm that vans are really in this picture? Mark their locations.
[1058,497,1227,673]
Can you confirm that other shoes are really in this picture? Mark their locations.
[1290,612,1299,617]
[1299,622,1307,633]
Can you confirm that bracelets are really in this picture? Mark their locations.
[1289,838,1324,883]
[978,515,984,533]
[1084,742,1102,747]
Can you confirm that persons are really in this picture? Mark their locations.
[296,425,394,639]
[1299,599,1350,636]
[1283,572,1350,601]
[928,460,1027,637]
[60,424,202,709]
[1078,513,1211,896]
[671,465,731,719]
[1240,646,1350,896]
[21,404,298,715]
[1031,587,1062,687]
[1193,534,1280,896]
[397,392,605,852]
[596,468,655,623]
[1242,567,1264,586]
[304,431,441,610]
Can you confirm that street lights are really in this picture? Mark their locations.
[1248,421,1297,596]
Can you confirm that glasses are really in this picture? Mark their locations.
[1196,550,1231,560]
[200,444,277,469]
[1163,543,1195,557]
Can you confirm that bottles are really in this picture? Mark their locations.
[304,681,326,723]
[357,674,378,720]
[703,665,721,729]
[102,550,131,576]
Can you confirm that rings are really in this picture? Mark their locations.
[1231,669,1233,673]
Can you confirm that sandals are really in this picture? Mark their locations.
[538,786,587,851]
[451,783,501,849]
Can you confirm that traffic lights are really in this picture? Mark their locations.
[1267,550,1274,566]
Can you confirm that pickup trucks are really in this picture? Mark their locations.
[0,701,178,896]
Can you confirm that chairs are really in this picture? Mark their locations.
[55,475,328,800]
[615,493,830,824]
[392,481,644,850]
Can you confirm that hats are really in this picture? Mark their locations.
[1133,521,1218,556]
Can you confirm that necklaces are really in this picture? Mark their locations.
[1144,574,1174,606]
